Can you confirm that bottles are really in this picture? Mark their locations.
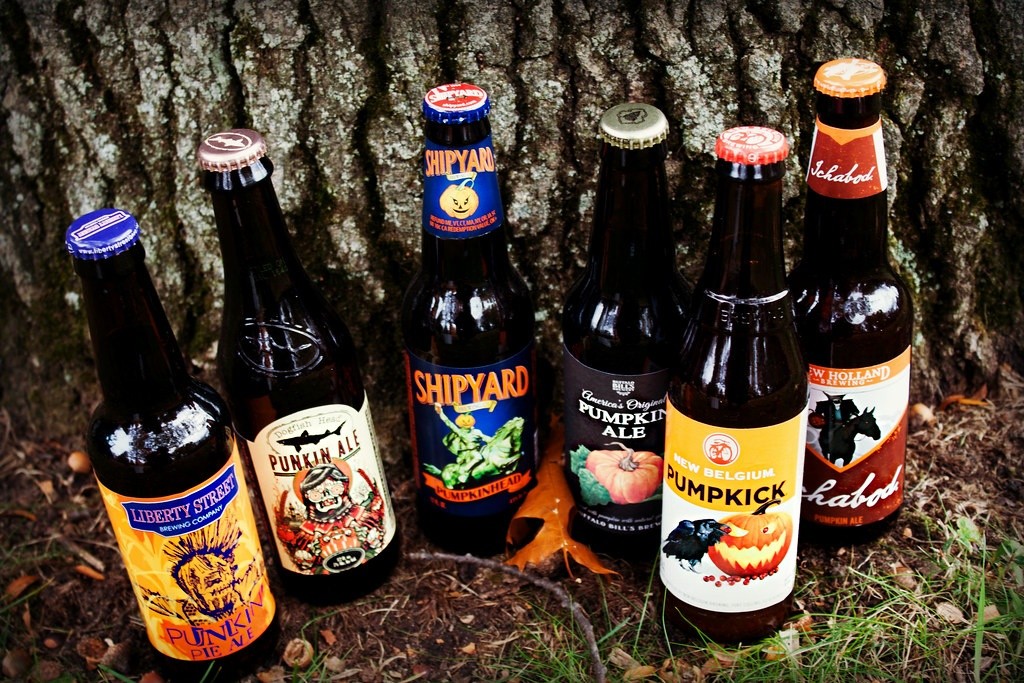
[410,84,548,561]
[196,126,402,608]
[63,208,282,683]
[564,102,692,556]
[780,56,911,553]
[657,125,807,643]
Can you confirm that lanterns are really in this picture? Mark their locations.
[707,499,794,578]
[439,179,479,220]
